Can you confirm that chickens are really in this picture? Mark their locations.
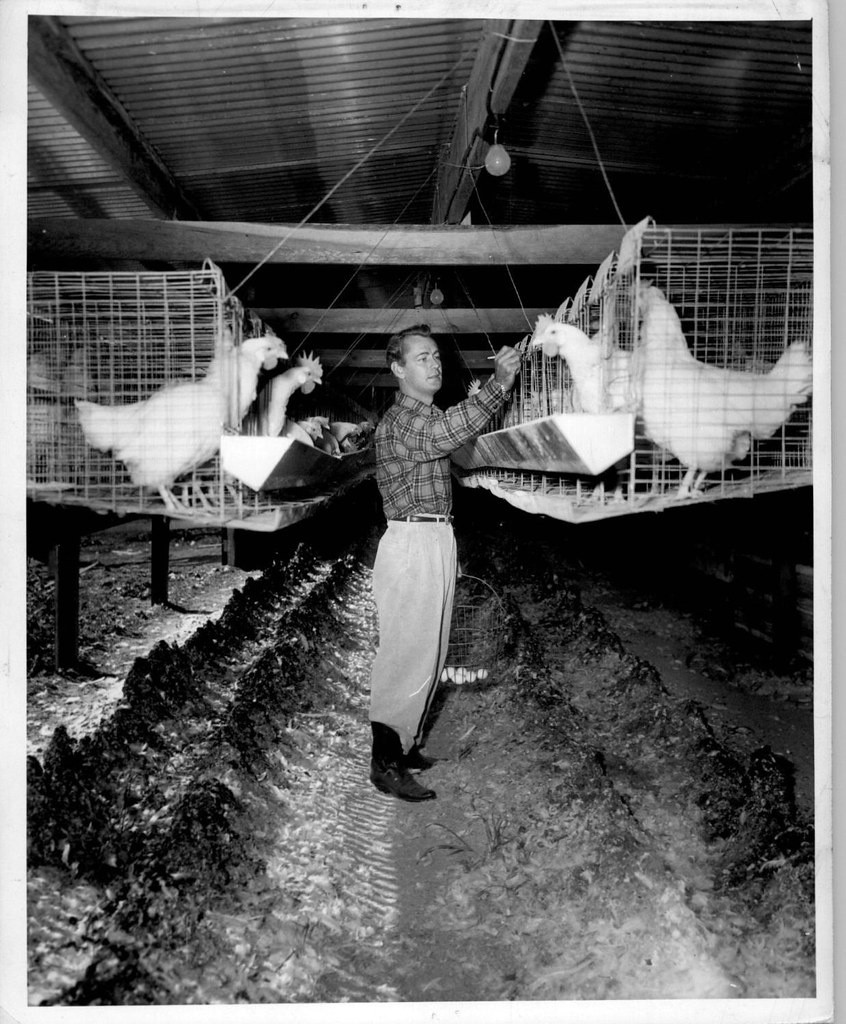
[466,378,482,397]
[223,349,323,509]
[292,414,376,455]
[73,336,288,511]
[533,312,675,504]
[627,279,812,498]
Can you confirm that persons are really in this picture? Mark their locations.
[368,322,523,802]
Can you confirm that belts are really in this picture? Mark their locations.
[390,515,454,523]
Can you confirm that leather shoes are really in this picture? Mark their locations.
[369,751,449,802]
